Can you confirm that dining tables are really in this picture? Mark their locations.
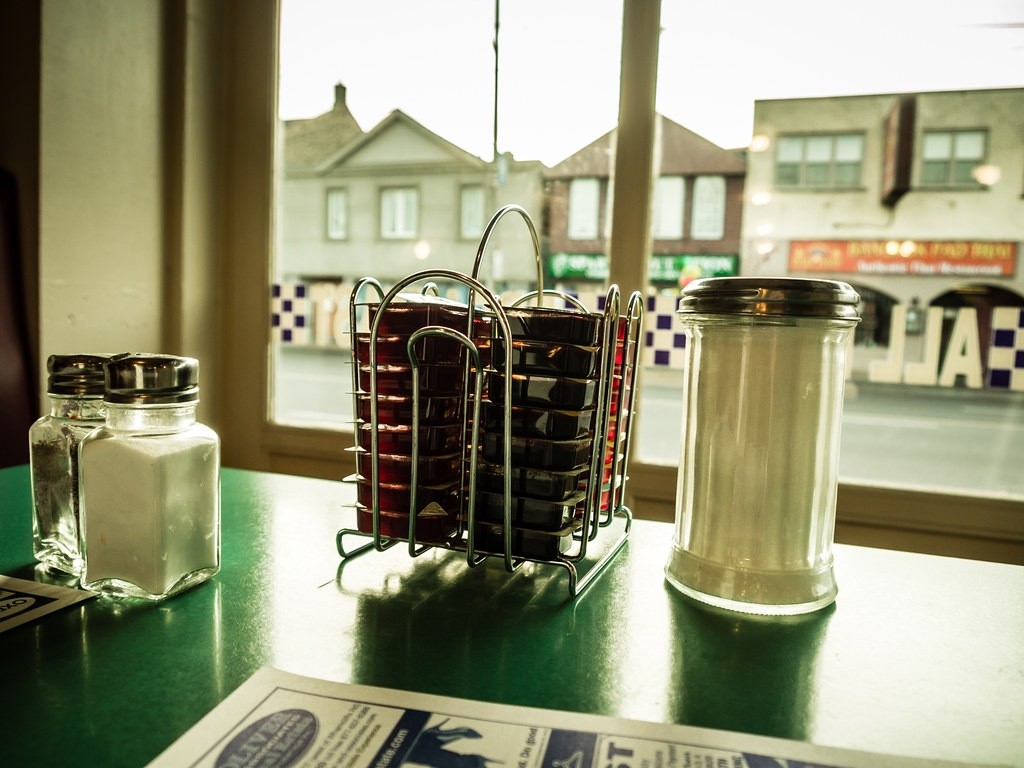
[0,466,1024,768]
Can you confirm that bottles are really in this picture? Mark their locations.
[28,353,107,575]
[77,353,222,604]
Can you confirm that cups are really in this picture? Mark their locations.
[663,275,864,616]
